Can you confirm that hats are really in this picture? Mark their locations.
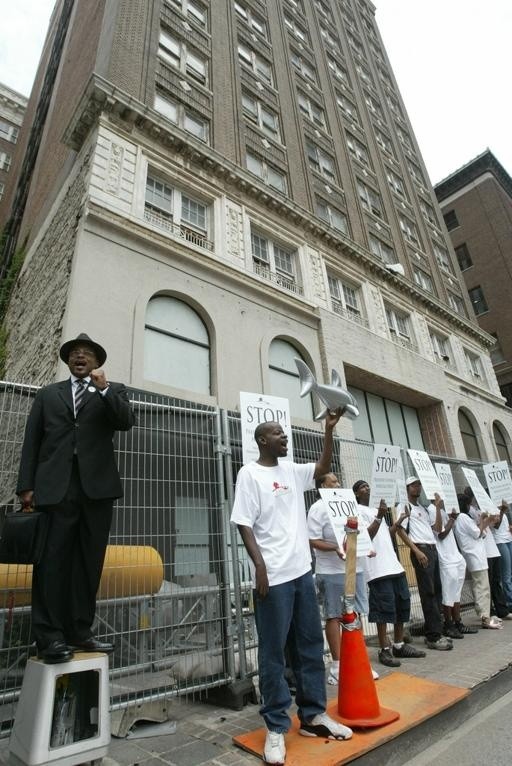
[405,476,420,486]
[60,334,106,369]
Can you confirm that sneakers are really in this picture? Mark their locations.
[328,667,339,685]
[393,644,425,657]
[378,649,400,667]
[370,667,380,680]
[299,711,353,740]
[425,612,512,650]
[263,729,286,765]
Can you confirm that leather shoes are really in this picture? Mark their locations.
[67,637,115,652]
[37,641,73,664]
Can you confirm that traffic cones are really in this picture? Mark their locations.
[325,602,397,726]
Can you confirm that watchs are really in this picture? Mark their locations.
[374,516,381,525]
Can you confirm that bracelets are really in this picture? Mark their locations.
[450,516,454,521]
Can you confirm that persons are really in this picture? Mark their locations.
[451,494,503,629]
[353,480,426,668]
[15,333,135,665]
[230,405,353,764]
[397,475,453,651]
[464,486,512,620]
[425,492,478,639]
[482,488,512,612]
[308,473,379,684]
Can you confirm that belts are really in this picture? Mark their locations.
[415,544,436,549]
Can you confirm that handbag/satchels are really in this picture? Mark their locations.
[1,503,47,564]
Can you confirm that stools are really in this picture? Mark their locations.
[8,653,109,766]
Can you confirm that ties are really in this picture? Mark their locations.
[75,378,87,413]
